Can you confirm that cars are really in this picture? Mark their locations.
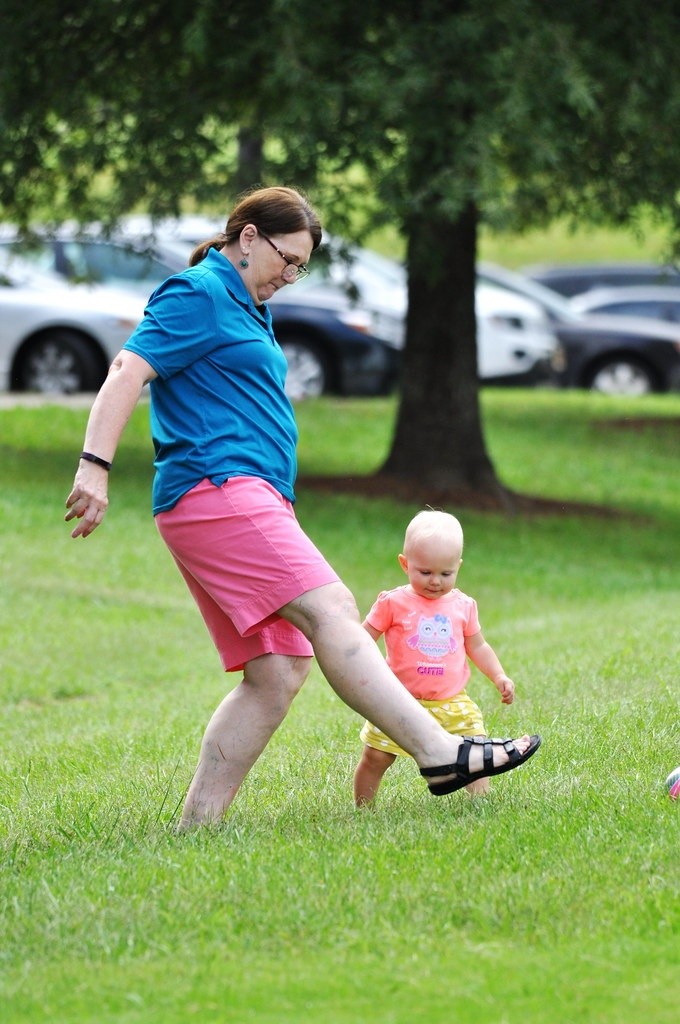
[0,205,680,395]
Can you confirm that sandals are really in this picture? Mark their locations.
[420,734,541,796]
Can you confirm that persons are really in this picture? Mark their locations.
[63,187,540,833]
[346,502,514,810]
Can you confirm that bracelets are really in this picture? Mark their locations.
[80,451,112,469]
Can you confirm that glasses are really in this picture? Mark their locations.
[255,226,310,282]
